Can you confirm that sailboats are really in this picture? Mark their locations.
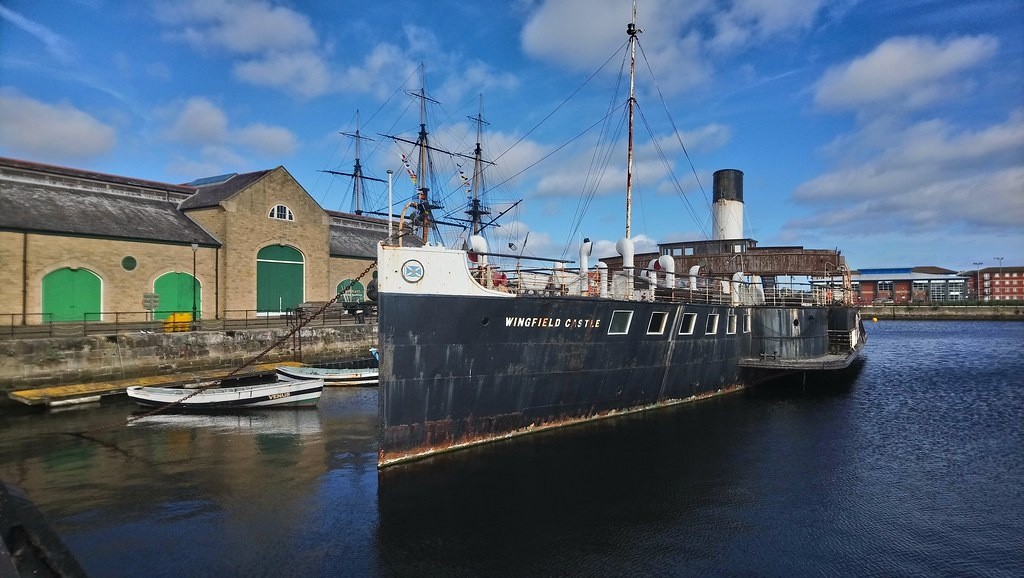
[376,0,869,469]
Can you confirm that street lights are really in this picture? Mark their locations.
[973,262,983,301]
[997,257,1004,302]
[190,243,198,331]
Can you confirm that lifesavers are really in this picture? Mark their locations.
[587,272,600,295]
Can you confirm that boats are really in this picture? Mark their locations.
[276,366,379,387]
[126,377,325,409]
